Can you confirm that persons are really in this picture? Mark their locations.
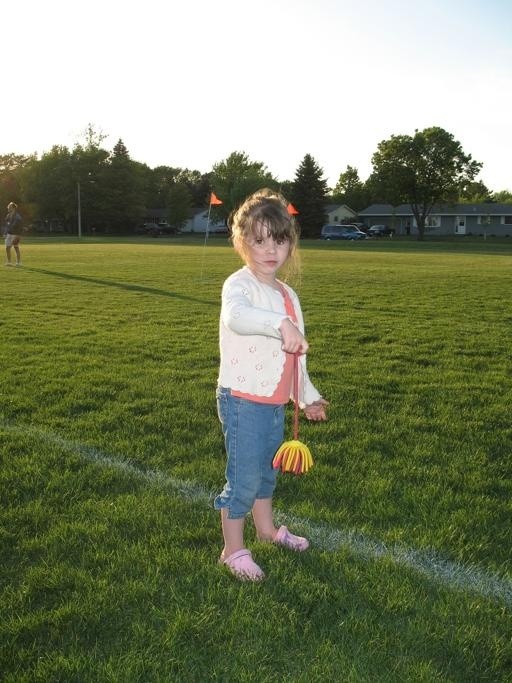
[212,185,332,584]
[2,201,24,268]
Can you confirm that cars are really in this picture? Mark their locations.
[152,222,178,235]
[216,223,229,232]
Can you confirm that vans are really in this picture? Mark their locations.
[320,224,367,240]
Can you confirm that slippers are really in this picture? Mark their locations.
[257,525,310,555]
[219,547,266,582]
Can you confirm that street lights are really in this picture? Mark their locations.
[76,179,96,238]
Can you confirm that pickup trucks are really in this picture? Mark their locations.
[367,223,395,236]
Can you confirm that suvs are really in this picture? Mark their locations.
[350,222,367,232]
